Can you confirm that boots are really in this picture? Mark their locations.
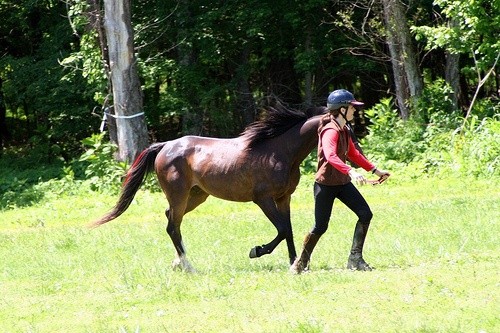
[347,221,372,272]
[290,231,321,275]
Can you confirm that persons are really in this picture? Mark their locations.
[289,89,391,275]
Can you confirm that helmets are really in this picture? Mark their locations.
[327,89,364,110]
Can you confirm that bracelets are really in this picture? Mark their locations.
[372,166,377,174]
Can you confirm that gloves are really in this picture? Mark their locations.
[348,168,367,187]
[375,170,391,178]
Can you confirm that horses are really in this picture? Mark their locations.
[86,101,368,273]
